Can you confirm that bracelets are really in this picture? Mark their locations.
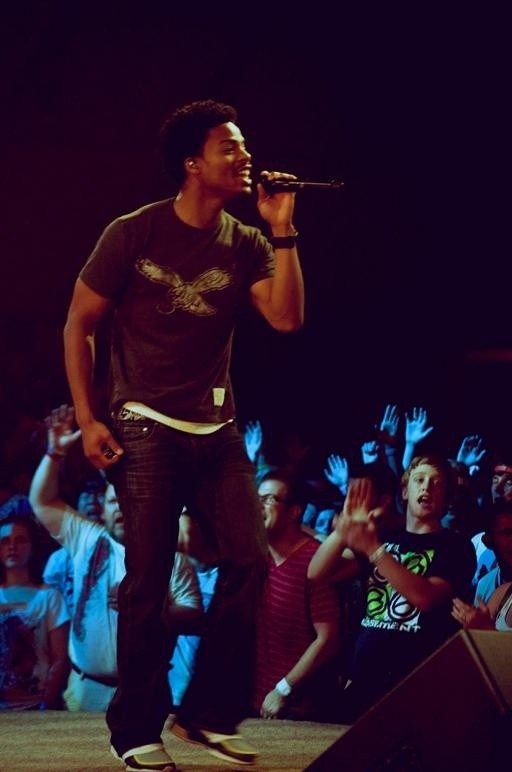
[268,231,301,252]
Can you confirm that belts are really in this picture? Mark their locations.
[71,663,117,687]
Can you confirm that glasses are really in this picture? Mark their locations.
[257,493,292,507]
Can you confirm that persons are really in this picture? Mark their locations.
[1,398,512,725]
[61,95,308,772]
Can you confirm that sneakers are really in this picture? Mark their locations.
[169,719,261,766]
[109,740,176,771]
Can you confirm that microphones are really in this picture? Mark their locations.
[250,173,344,197]
